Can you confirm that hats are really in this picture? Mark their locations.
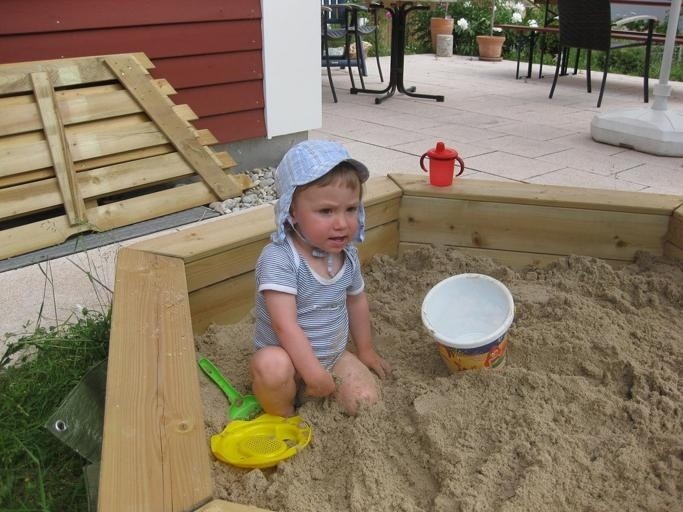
[270,140,368,251]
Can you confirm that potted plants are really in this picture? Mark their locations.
[476,0,506,61]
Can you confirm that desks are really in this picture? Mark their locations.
[534,0,683,78]
[350,0,443,104]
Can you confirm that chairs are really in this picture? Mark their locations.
[321,3,383,103]
[549,0,658,106]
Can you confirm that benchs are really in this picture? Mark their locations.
[494,24,683,79]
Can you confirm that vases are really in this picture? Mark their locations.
[430,17,454,54]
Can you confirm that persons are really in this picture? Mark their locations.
[250,136,394,419]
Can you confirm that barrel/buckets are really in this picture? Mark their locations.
[420,272,517,372]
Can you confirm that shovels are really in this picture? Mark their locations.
[198,355,265,424]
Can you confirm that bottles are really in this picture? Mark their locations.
[419,141,464,186]
